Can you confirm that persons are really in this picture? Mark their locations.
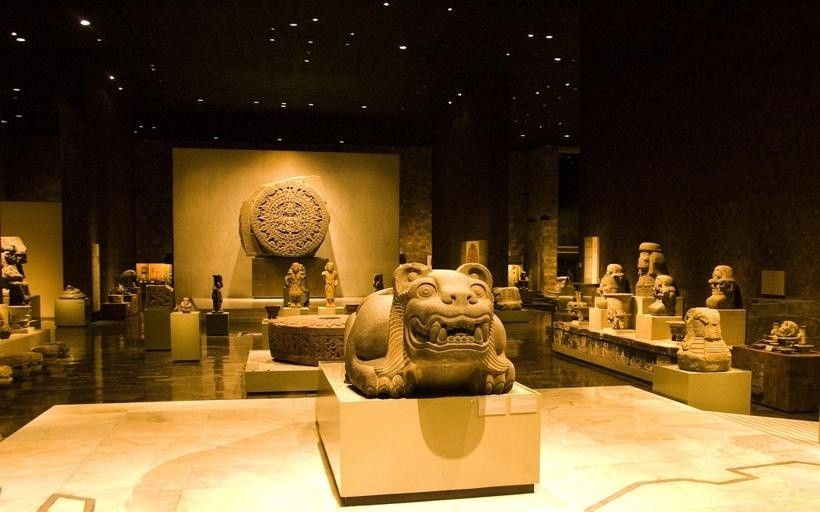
[322,262,338,306]
[284,262,308,307]
